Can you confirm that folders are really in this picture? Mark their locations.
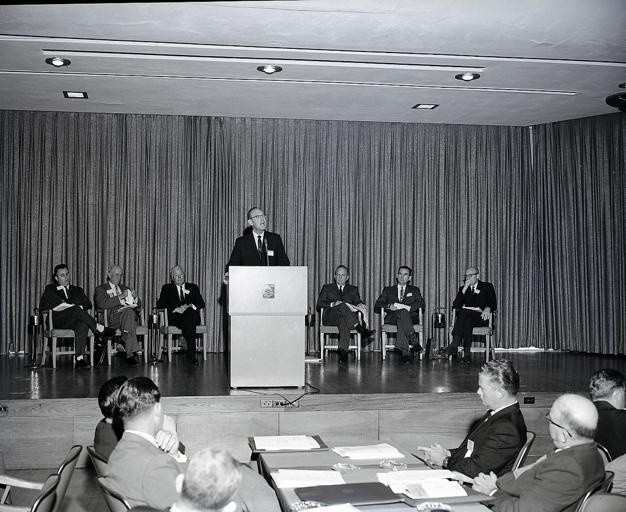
[294,482,404,508]
[247,433,329,453]
[397,485,497,507]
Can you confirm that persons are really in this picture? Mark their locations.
[376,265,427,356]
[472,393,606,512]
[589,368,626,462]
[105,376,281,512]
[129,446,237,512]
[94,267,145,360]
[94,375,128,459]
[427,358,528,479]
[155,265,205,367]
[440,267,497,363]
[40,264,121,371]
[316,265,376,362]
[224,208,290,274]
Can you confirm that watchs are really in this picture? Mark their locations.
[442,456,450,468]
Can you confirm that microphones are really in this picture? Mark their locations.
[264,239,269,265]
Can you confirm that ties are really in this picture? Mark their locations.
[471,286,475,294]
[257,235,262,258]
[180,285,184,300]
[64,286,69,297]
[115,285,119,295]
[339,286,342,294]
[400,287,403,301]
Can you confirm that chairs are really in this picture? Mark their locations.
[449,307,491,361]
[94,477,136,512]
[33,308,95,369]
[96,310,148,365]
[0,445,79,512]
[318,307,361,362]
[379,306,423,360]
[86,446,117,476]
[511,431,536,471]
[569,469,617,512]
[155,308,208,362]
[0,475,55,512]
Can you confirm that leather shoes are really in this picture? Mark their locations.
[338,356,347,363]
[458,357,471,364]
[101,327,115,338]
[413,343,423,352]
[189,357,199,366]
[361,329,376,340]
[75,359,91,369]
[126,357,139,368]
[400,355,411,364]
[440,345,458,354]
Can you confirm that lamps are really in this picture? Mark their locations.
[257,65,282,74]
[44,56,72,69]
[456,73,480,83]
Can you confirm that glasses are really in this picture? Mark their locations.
[464,272,478,277]
[249,213,266,219]
[544,412,572,438]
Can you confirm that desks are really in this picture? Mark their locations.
[224,264,308,389]
[254,430,494,512]
